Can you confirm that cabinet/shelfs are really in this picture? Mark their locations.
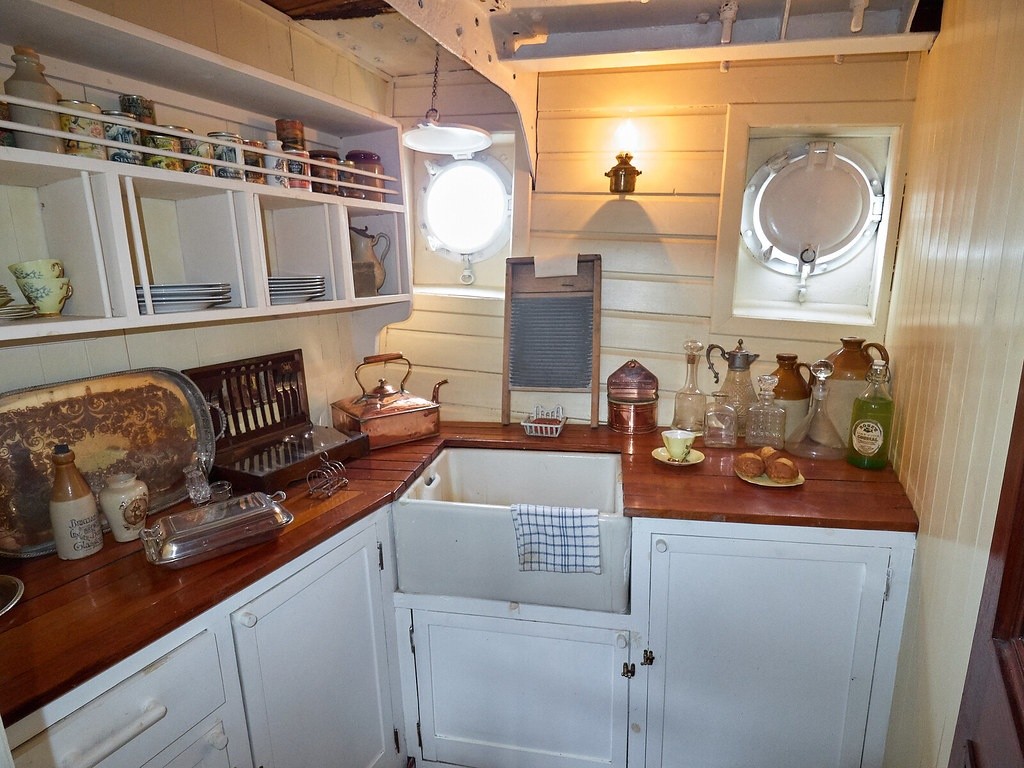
[0,0,416,344]
[392,590,631,768]
[1,493,411,768]
[622,426,919,767]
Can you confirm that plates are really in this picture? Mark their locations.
[735,468,805,486]
[0,283,37,319]
[268,274,326,304]
[652,447,705,466]
[135,281,232,314]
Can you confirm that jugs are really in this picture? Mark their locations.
[704,339,759,437]
[349,225,390,295]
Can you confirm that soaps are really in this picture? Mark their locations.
[528,418,561,434]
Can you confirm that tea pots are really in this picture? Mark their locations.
[329,351,450,449]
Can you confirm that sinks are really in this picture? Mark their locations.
[398,447,632,615]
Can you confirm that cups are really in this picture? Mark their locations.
[660,430,696,462]
[352,260,378,299]
[183,465,210,505]
[8,259,64,279]
[209,481,232,503]
[15,278,73,320]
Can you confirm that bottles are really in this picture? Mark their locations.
[4,44,66,153]
[782,360,848,459]
[48,444,104,561]
[847,360,895,470]
[669,340,708,436]
[745,375,785,451]
[264,140,290,187]
[807,336,889,449]
[704,392,737,448]
[99,473,149,543]
[760,353,815,444]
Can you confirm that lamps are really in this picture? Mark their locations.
[605,121,643,194]
[401,118,516,285]
